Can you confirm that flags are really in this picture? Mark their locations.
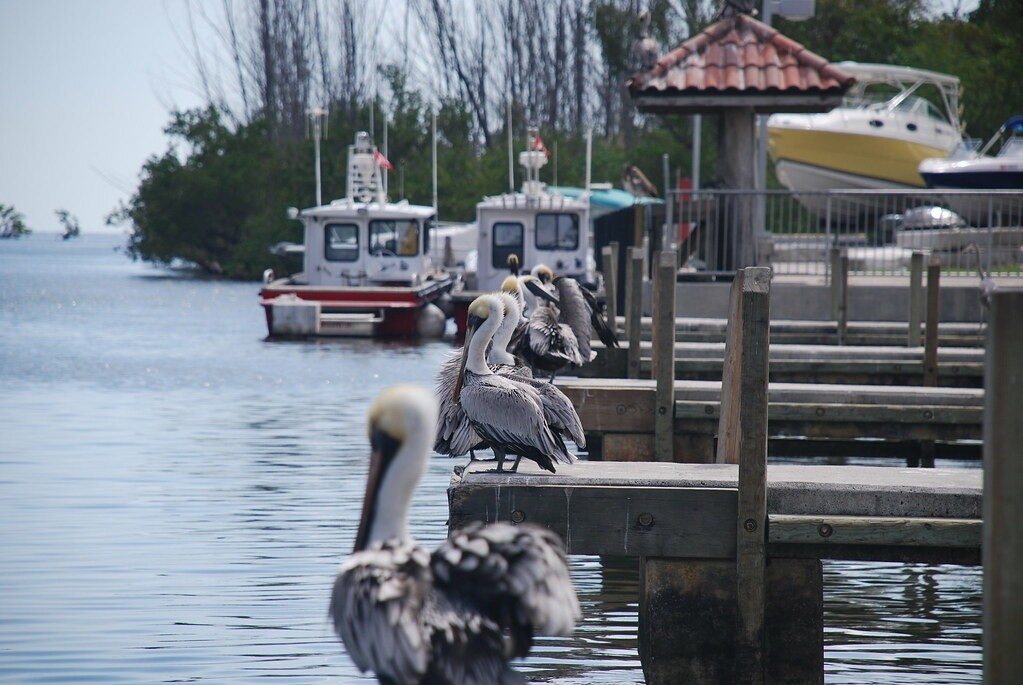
[529,136,549,157]
[371,152,394,168]
[675,172,699,203]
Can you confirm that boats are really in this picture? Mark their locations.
[765,60,1023,228]
[258,106,694,341]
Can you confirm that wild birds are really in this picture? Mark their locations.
[329,264,621,685]
